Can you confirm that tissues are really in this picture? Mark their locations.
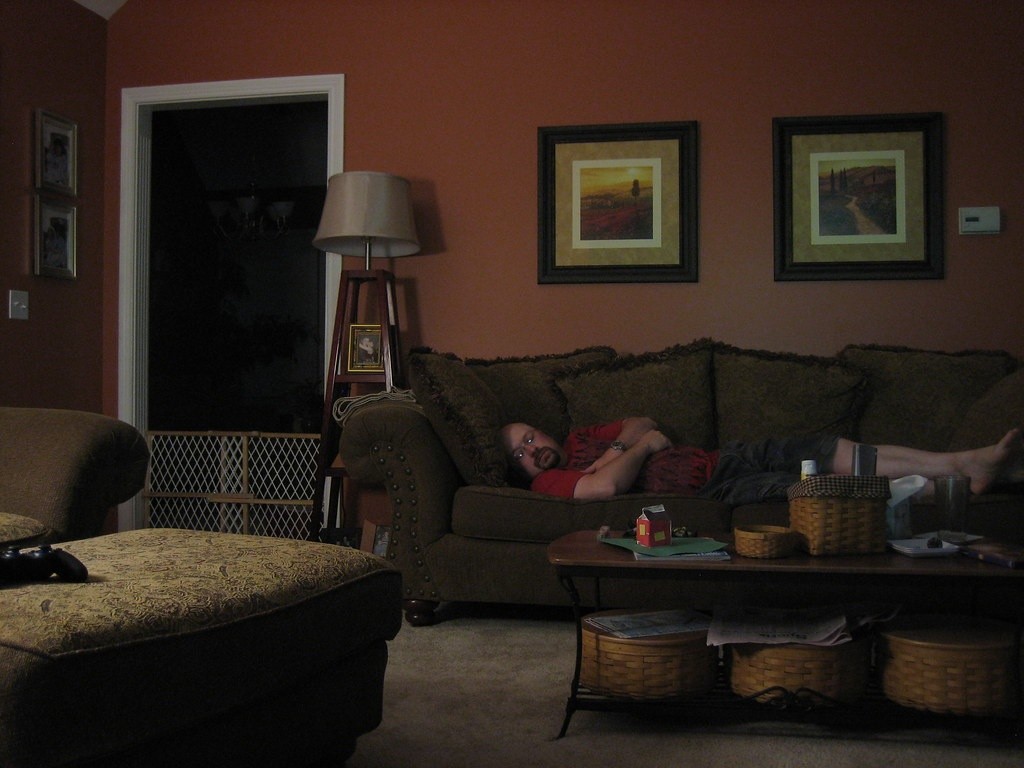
[886,474,941,540]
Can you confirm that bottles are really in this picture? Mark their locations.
[800,459,819,481]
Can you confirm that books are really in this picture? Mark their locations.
[633,536,730,561]
[961,543,1024,569]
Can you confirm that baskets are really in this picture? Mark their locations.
[733,524,794,559]
[879,626,1018,716]
[788,475,889,554]
[580,609,719,702]
[724,626,874,708]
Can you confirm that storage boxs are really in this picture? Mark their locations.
[636,505,672,546]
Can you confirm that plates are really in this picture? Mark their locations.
[887,531,984,558]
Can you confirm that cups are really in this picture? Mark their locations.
[933,474,971,543]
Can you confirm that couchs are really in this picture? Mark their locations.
[0,408,149,548]
[337,338,1024,629]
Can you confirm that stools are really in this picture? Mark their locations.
[0,528,404,768]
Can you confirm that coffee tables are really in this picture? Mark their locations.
[543,527,1024,739]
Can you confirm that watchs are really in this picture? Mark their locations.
[610,441,627,452]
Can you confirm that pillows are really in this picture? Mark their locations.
[463,345,618,446]
[408,347,510,487]
[837,344,1015,452]
[711,341,876,454]
[547,339,715,449]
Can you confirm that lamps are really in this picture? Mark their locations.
[313,172,420,529]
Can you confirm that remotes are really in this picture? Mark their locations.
[852,444,878,477]
[625,527,699,538]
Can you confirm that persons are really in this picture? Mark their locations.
[494,417,1024,504]
[358,336,376,363]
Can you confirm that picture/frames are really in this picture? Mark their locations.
[535,121,701,284]
[767,111,949,283]
[32,194,79,282]
[34,108,81,197]
[372,525,391,557]
[344,324,388,373]
